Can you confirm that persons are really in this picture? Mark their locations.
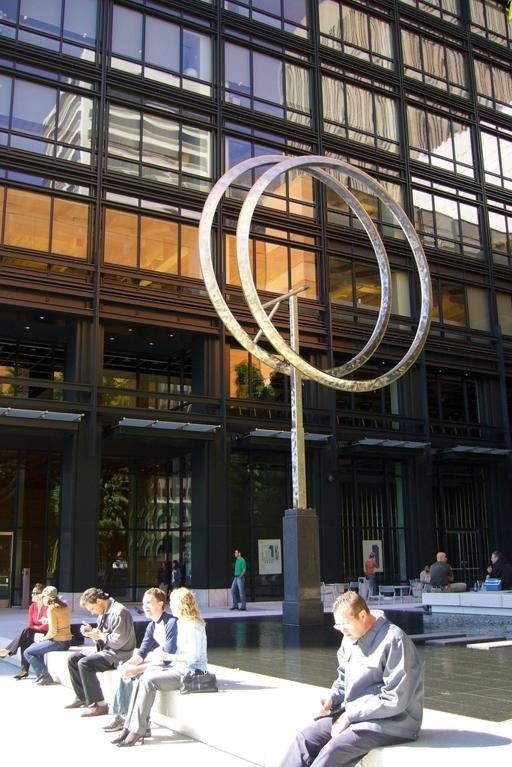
[171,560,181,589]
[430,552,467,593]
[112,586,209,746]
[281,590,425,767]
[420,564,431,585]
[0,582,48,678]
[64,587,135,716]
[365,552,377,592]
[22,585,72,685]
[230,548,247,610]
[486,551,511,590]
[157,561,169,602]
[103,587,178,733]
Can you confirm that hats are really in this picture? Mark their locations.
[42,586,58,599]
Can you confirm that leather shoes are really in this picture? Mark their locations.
[64,700,88,708]
[80,703,109,718]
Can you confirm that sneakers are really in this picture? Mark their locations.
[102,719,152,737]
[229,606,247,612]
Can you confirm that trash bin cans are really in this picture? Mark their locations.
[358,577,368,602]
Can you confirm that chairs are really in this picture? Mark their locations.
[320,576,424,606]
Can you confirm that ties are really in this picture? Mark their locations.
[96,614,108,651]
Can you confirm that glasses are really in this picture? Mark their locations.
[333,621,355,631]
[32,591,42,595]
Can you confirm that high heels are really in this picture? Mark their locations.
[34,674,53,686]
[111,727,145,748]
[14,672,29,680]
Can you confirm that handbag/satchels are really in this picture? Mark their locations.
[178,668,219,695]
[70,632,84,646]
